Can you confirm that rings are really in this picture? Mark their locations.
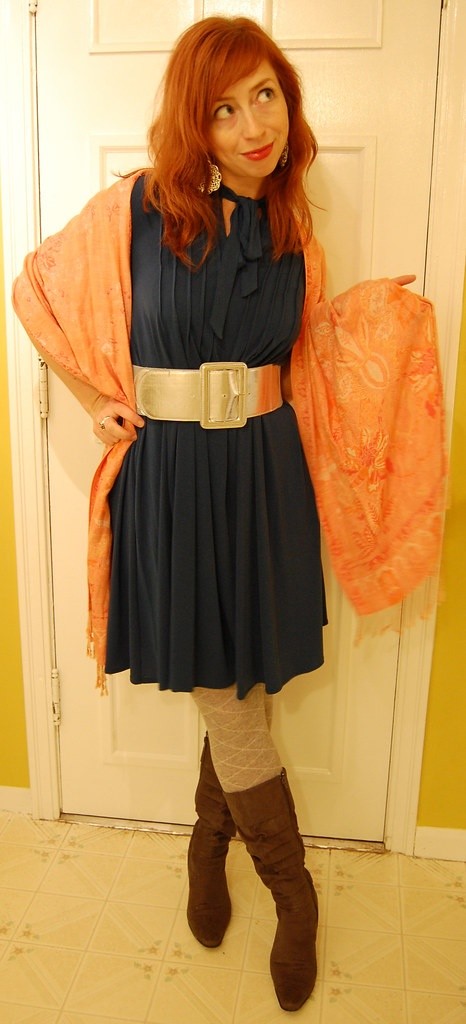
[99,416,112,430]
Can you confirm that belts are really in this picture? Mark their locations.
[133,361,283,429]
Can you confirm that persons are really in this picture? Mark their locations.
[11,18,450,1012]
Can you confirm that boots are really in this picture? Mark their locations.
[221,767,318,1011]
[187,730,237,948]
